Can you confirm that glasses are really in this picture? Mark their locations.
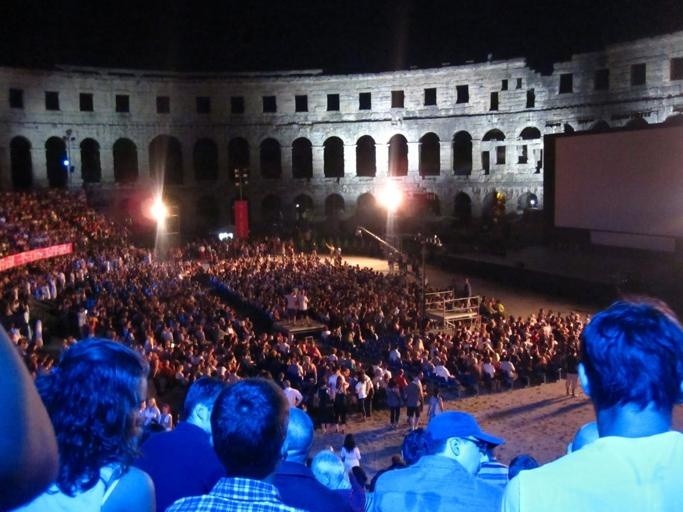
[462,437,487,456]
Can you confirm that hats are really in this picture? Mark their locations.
[424,412,505,449]
